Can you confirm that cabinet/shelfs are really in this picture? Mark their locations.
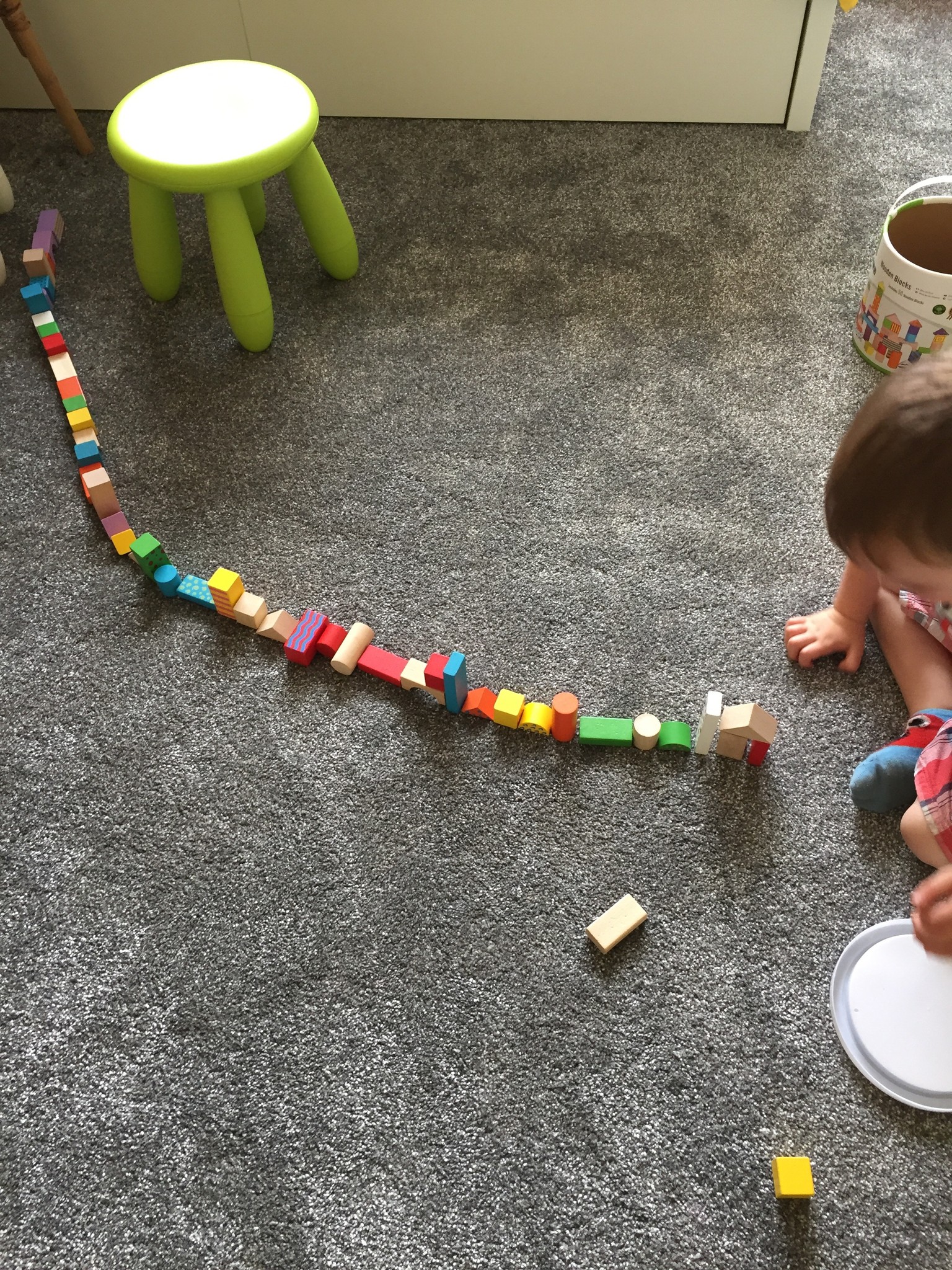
[0,0,837,132]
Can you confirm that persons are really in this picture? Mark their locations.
[782,352,952,956]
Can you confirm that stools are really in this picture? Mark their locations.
[107,59,359,351]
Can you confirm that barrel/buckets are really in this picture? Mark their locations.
[852,176,952,375]
